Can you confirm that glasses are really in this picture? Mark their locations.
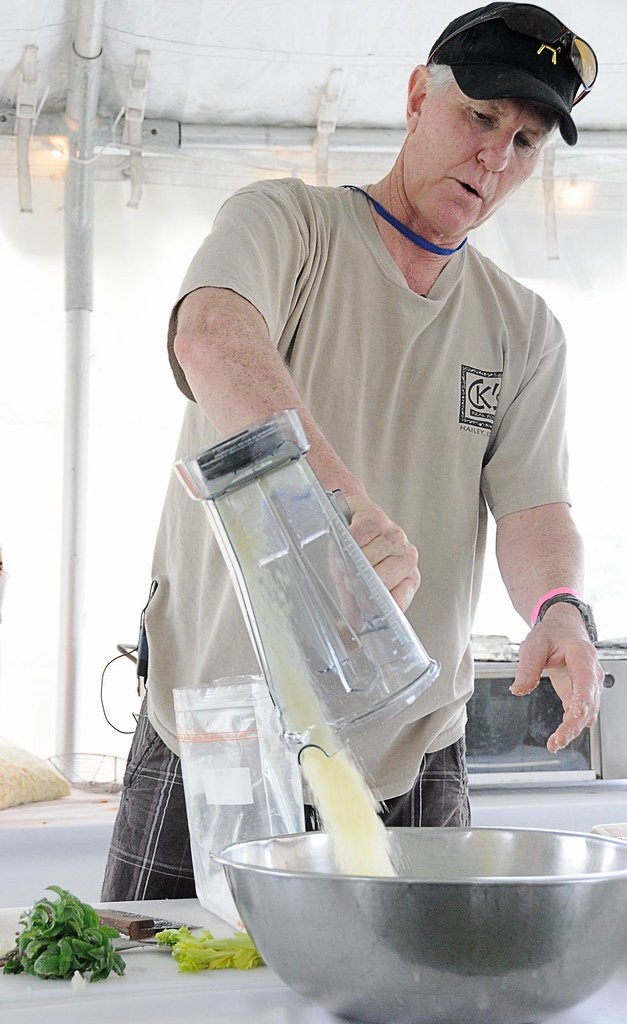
[426,5,599,109]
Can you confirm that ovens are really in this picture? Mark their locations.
[464,645,627,794]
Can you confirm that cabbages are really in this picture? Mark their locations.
[154,924,265,973]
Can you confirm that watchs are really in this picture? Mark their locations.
[534,595,597,645]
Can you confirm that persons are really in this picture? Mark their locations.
[92,3,604,900]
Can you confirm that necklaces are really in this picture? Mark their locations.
[340,184,469,257]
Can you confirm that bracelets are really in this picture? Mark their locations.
[528,586,581,628]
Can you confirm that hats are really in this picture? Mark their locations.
[425,2,584,146]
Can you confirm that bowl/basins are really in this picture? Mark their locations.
[209,826,627,1024]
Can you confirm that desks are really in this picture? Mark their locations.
[0,736,627,912]
[0,888,627,1024]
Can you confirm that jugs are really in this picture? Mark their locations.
[168,407,444,762]
